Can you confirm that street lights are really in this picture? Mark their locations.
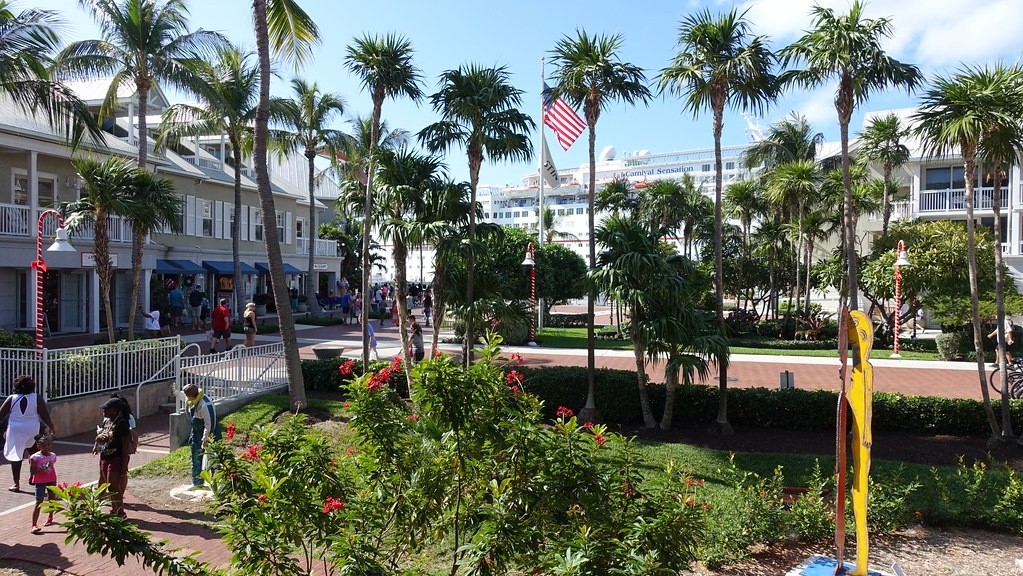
[520,241,538,347]
[34,210,78,358]
[889,239,913,353]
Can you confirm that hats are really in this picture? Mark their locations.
[98,397,122,409]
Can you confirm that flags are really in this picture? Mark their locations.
[538,135,561,189]
[544,82,587,151]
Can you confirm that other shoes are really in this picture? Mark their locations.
[225,346,238,351]
[109,510,128,523]
[8,486,19,492]
[397,324,399,327]
[30,526,41,534]
[187,484,204,491]
[380,323,384,326]
[44,522,52,526]
[210,348,217,354]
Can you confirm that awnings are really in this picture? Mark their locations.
[157,259,207,274]
[203,260,259,274]
[256,262,302,274]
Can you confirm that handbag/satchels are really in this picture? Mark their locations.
[0,413,9,448]
[409,347,413,358]
[127,429,138,454]
[202,449,217,470]
[207,332,214,344]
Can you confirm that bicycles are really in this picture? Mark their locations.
[988,356,1023,401]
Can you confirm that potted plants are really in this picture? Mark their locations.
[297,295,307,312]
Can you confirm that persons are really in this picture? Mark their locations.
[0,376,56,491]
[316,289,362,327]
[406,282,432,327]
[368,283,399,326]
[408,316,425,363]
[988,315,1014,368]
[912,299,924,333]
[142,304,162,337]
[210,299,232,353]
[358,316,378,359]
[29,438,57,532]
[243,303,257,345]
[92,394,135,522]
[287,286,298,312]
[184,384,221,490]
[189,286,209,331]
[170,285,185,326]
